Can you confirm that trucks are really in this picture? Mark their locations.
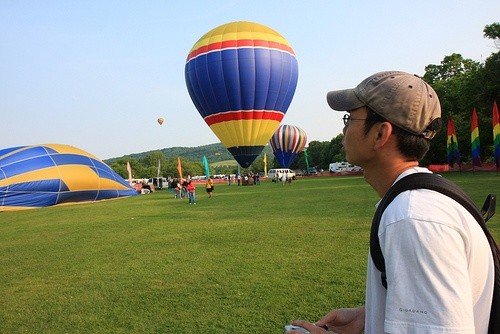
[328,161,364,176]
[124,177,169,190]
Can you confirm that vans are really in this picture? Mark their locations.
[268,168,296,182]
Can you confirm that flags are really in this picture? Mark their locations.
[157,159,161,177]
[178,157,183,178]
[127,162,132,184]
[203,155,209,179]
[471,108,482,166]
[447,116,461,168]
[493,101,500,165]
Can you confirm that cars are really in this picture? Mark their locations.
[306,168,317,175]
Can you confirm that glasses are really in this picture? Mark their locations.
[342,114,366,127]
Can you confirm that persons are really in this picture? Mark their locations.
[321,168,324,174]
[228,175,230,186]
[206,176,214,198]
[231,176,235,184]
[290,70,494,334]
[330,167,335,176]
[168,179,197,205]
[272,170,296,186]
[237,173,260,186]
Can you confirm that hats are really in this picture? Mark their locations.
[327,71,441,139]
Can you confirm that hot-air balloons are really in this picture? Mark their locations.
[184,20,299,186]
[269,124,307,168]
[157,118,164,125]
[0,144,142,212]
